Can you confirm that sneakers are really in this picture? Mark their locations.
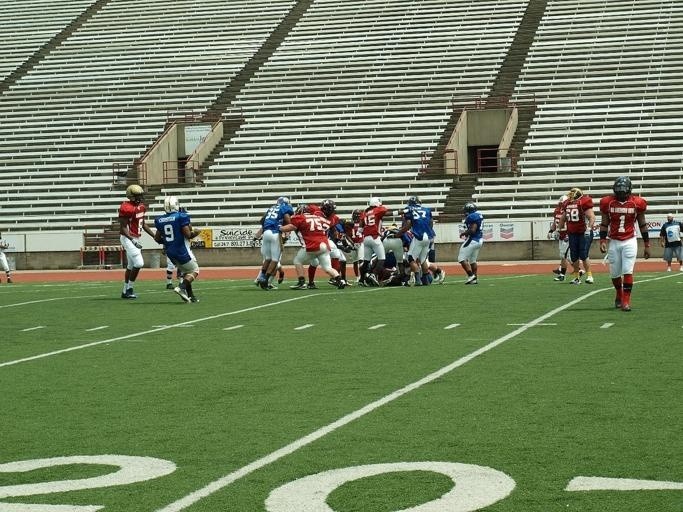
[167,283,199,303]
[615,298,622,307]
[679,265,683,272]
[465,274,476,284]
[121,288,136,298]
[358,270,446,287]
[667,266,671,272]
[622,304,631,311]
[255,271,352,290]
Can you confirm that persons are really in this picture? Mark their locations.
[547,176,683,311]
[1,232,14,283]
[119,185,200,303]
[250,196,483,290]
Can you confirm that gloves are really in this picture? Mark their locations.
[584,228,591,239]
[132,239,143,249]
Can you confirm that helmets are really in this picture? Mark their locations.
[126,184,144,206]
[409,197,419,206]
[164,196,180,213]
[277,197,289,205]
[558,187,583,207]
[613,176,631,202]
[352,209,365,223]
[553,269,593,285]
[295,205,312,215]
[321,200,337,218]
[463,202,477,217]
[370,197,383,207]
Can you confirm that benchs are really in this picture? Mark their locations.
[0,0,683,234]
